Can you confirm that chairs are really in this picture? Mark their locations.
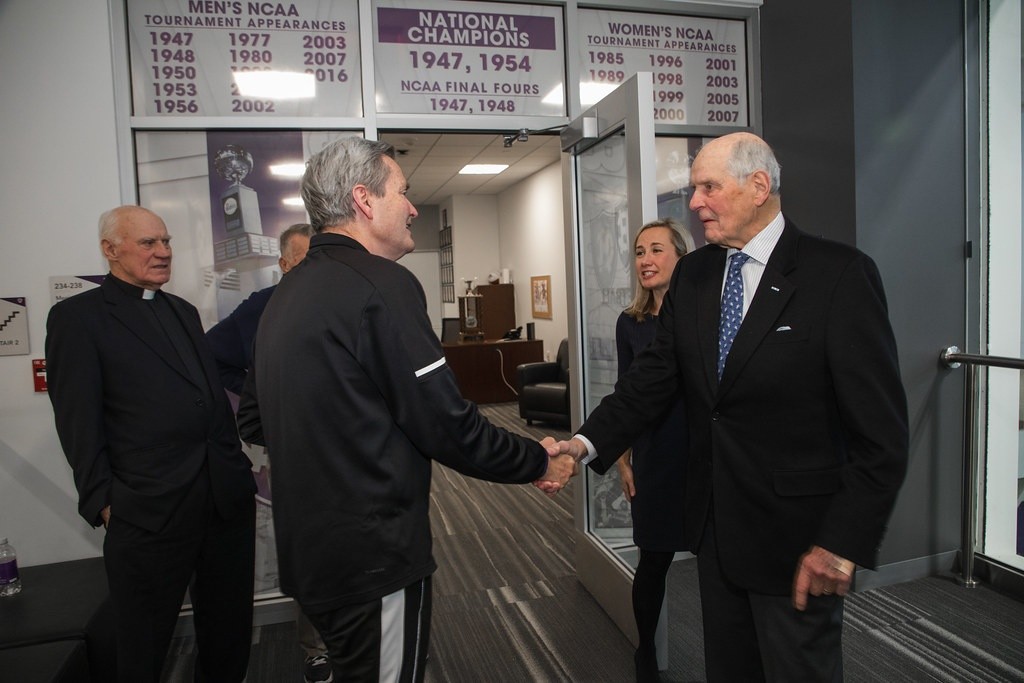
[441,317,463,342]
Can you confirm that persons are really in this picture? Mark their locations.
[539,132,907,682]
[201,224,335,683]
[617,222,701,682]
[238,139,576,682]
[50,204,256,683]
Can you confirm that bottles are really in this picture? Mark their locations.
[0,537,22,597]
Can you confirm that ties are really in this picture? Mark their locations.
[718,253,750,384]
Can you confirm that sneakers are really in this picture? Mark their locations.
[303,649,332,683]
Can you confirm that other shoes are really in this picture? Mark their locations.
[634,645,661,683]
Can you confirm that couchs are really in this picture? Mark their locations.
[515,335,572,427]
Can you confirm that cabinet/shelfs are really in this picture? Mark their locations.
[472,283,516,340]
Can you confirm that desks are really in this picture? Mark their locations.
[440,340,544,405]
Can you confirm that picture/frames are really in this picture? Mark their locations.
[531,275,553,319]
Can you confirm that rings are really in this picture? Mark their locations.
[824,590,832,594]
